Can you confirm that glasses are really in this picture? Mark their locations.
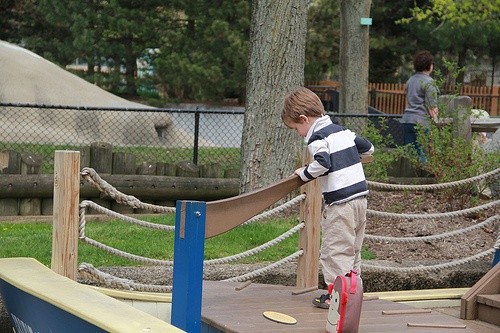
[431,61,435,66]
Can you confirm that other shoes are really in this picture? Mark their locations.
[313,292,332,309]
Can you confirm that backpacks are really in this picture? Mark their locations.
[326,270,363,333]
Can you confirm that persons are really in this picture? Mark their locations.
[401,51,447,174]
[281,87,375,308]
